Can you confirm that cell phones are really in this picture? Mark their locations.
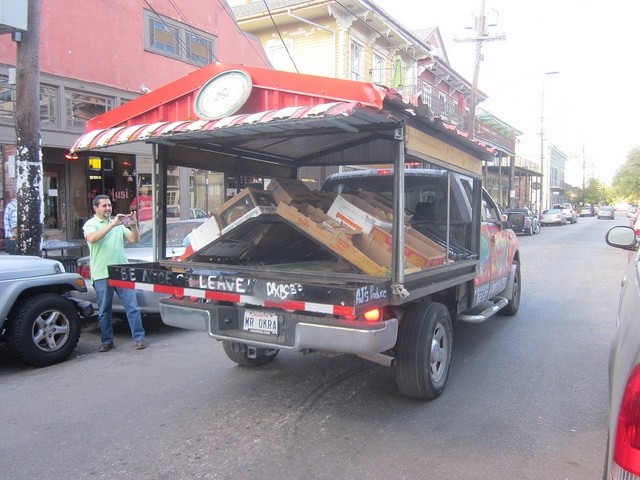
[121,216,131,224]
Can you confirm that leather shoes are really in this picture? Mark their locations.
[98,343,115,353]
[136,342,146,349]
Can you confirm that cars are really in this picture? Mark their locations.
[539,210,566,225]
[74,218,221,314]
[165,205,207,219]
[628,208,640,266]
[627,206,638,218]
[597,205,615,220]
[605,224,640,479]
[580,207,594,216]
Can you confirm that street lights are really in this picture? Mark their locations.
[539,70,559,217]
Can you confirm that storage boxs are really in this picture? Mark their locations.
[327,193,397,235]
[369,225,446,269]
[275,202,355,249]
[187,215,220,252]
[217,185,275,236]
[330,231,421,281]
[359,190,414,223]
[266,170,315,204]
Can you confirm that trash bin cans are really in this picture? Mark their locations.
[42,245,85,273]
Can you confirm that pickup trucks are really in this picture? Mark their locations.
[159,169,524,399]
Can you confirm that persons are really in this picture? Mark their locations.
[82,195,147,352]
[129,184,153,222]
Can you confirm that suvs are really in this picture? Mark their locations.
[502,208,541,235]
[552,204,577,224]
[0,249,94,368]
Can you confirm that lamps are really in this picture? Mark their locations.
[122,165,129,177]
[126,173,134,183]
[132,169,137,178]
